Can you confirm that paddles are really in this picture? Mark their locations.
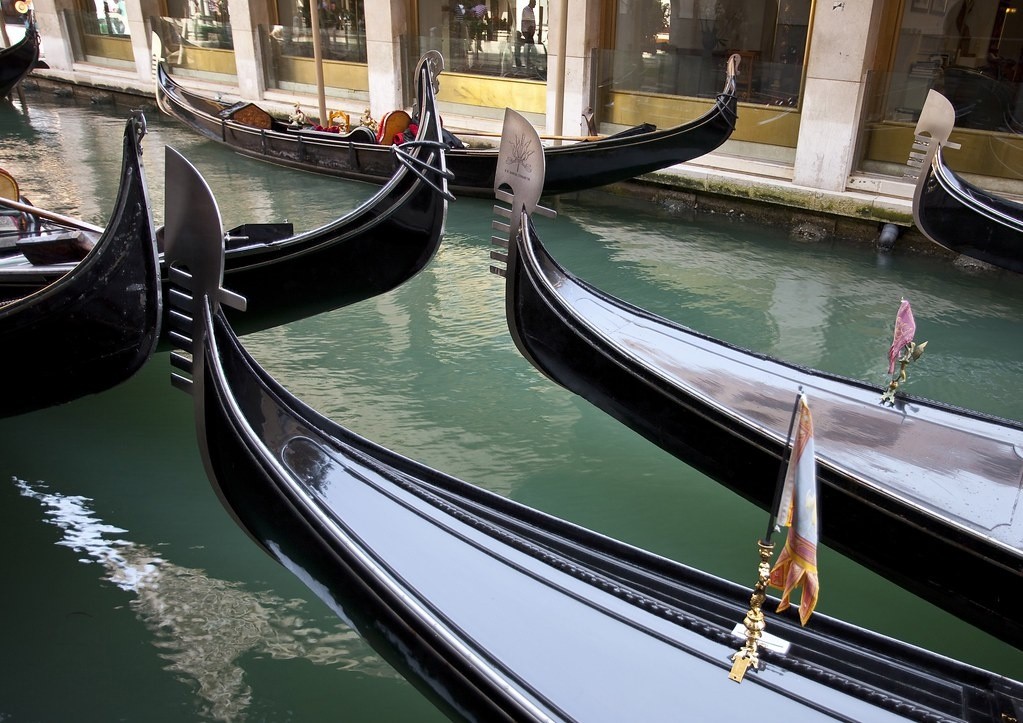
[224,222,295,250]
[0,197,166,253]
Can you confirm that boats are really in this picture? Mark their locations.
[488,105,1023,655]
[0,47,454,353]
[905,86,1023,276]
[162,143,1023,723]
[1,0,52,104]
[0,104,161,415]
[155,49,740,200]
